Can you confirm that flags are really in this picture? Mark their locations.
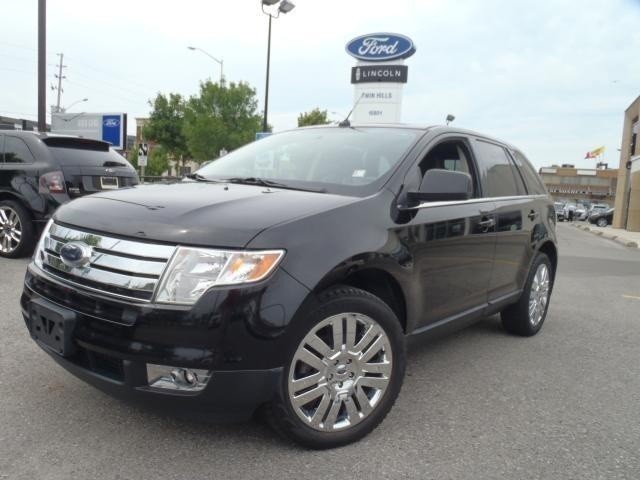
[591,147,605,158]
[585,152,596,160]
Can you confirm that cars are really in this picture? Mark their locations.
[552,199,614,228]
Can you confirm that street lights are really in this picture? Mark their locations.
[187,46,225,89]
[260,0,296,132]
[61,99,89,113]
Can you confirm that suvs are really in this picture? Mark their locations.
[0,130,140,258]
[16,86,559,454]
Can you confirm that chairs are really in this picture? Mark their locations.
[406,165,472,200]
